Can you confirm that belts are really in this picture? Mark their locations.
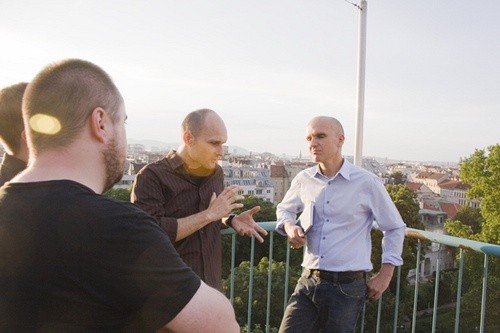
[311,270,365,280]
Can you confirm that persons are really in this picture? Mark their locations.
[275,115,406,333]
[0,82,29,187]
[130,108,268,295]
[0,59,240,333]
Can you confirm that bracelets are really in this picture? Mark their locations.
[225,214,235,227]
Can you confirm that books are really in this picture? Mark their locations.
[290,201,314,250]
